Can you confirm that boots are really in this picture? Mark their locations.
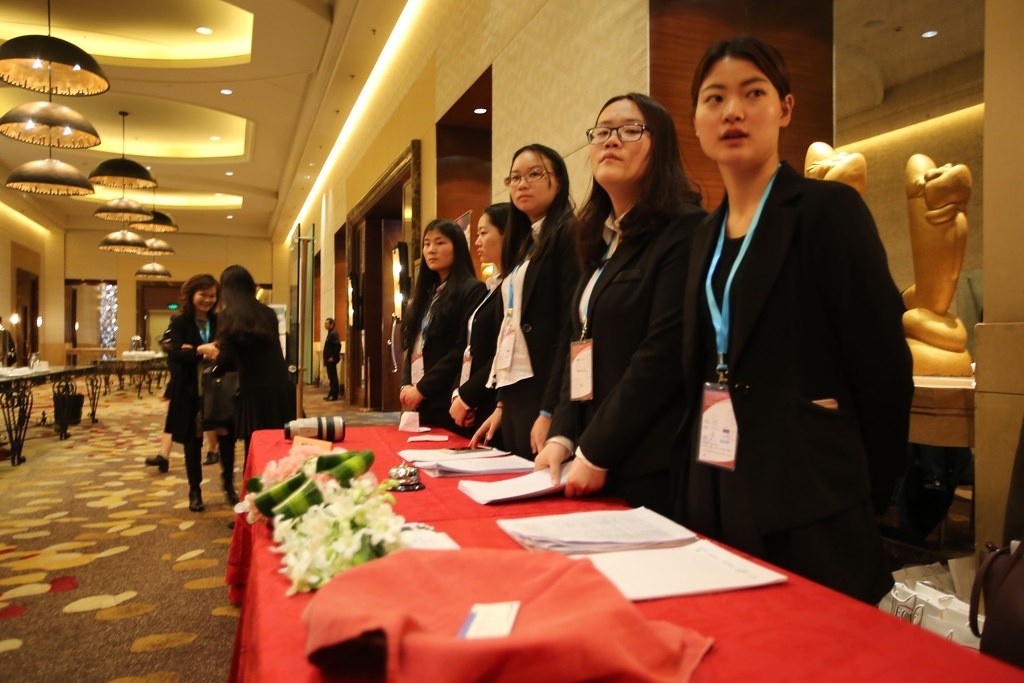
[216,433,239,504]
[184,437,204,511]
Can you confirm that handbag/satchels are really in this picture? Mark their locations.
[890,555,985,649]
[970,540,1024,667]
[200,364,235,423]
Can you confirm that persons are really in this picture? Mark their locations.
[681,36,914,597]
[468,144,580,460]
[323,318,341,400]
[143,265,294,511]
[448,202,512,442]
[533,94,708,520]
[400,219,487,432]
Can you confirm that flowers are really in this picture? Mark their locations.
[266,472,408,597]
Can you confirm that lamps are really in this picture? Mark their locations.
[128,190,179,280]
[0,0,110,197]
[87,111,158,254]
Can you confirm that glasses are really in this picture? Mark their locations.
[503,169,559,187]
[586,124,650,145]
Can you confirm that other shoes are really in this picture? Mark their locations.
[323,394,337,400]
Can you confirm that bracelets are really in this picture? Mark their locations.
[400,386,404,391]
[497,405,502,408]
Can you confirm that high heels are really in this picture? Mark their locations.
[145,455,169,473]
[203,451,219,465]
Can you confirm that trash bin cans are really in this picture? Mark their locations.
[53,393,84,425]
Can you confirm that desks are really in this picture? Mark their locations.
[222,423,1024,683]
[0,363,102,467]
[89,354,169,400]
